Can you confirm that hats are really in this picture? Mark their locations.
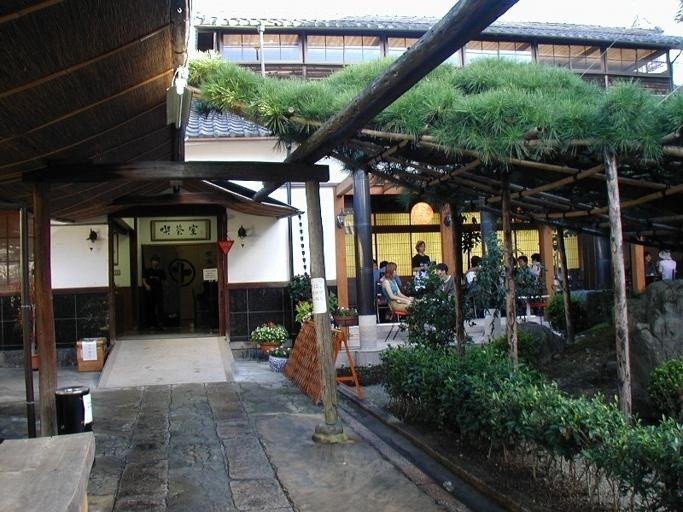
[658,249,671,260]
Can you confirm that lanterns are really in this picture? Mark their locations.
[411,202,434,226]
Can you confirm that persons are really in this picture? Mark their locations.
[373,239,542,321]
[644,249,677,282]
[142,255,168,333]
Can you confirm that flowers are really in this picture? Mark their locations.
[266,345,292,357]
[250,322,289,343]
[334,307,357,316]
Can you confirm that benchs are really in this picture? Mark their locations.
[0,431,96,511]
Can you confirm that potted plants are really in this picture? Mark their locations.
[12,275,39,370]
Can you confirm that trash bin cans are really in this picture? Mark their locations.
[54,386,96,468]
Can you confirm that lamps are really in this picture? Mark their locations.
[238,225,247,247]
[88,230,97,251]
[337,208,346,228]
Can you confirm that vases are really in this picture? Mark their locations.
[259,342,281,354]
[269,356,288,372]
[335,316,358,326]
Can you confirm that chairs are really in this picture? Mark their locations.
[377,282,432,342]
